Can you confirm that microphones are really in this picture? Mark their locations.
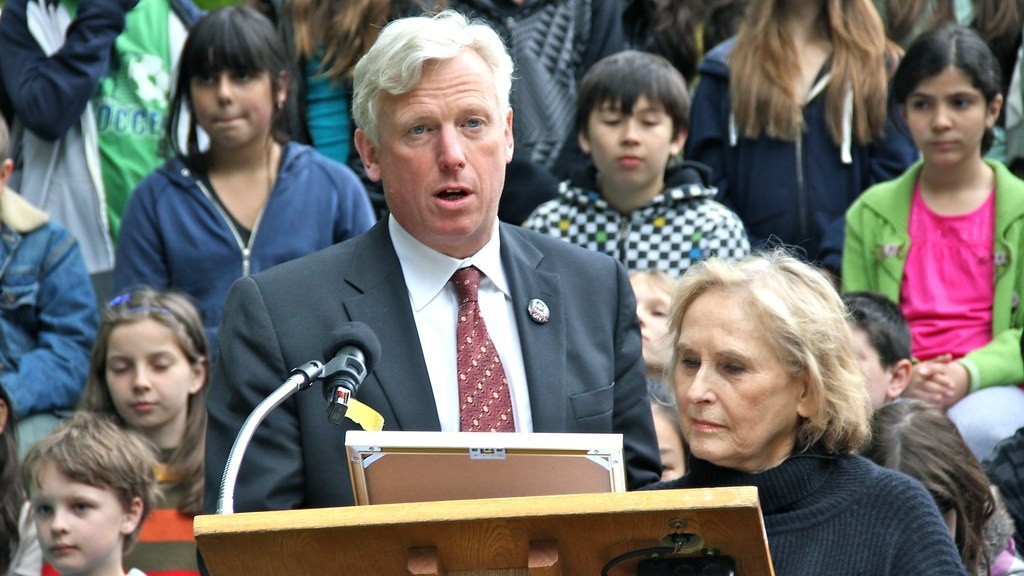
[322,321,382,427]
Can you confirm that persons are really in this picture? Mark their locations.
[0,0,1024,576]
[839,21,1024,467]
[630,247,974,576]
[113,7,380,360]
[22,411,163,576]
[201,10,664,515]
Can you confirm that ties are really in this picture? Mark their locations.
[450,266,514,432]
